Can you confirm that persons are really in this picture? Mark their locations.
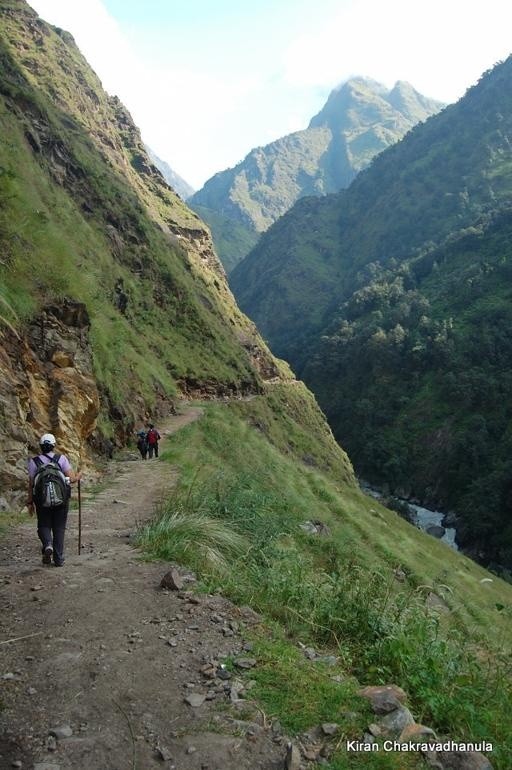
[146,425,161,459]
[137,432,149,459]
[26,434,82,566]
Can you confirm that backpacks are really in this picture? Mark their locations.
[148,430,157,445]
[32,453,71,511]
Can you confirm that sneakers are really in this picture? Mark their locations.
[55,560,65,567]
[43,544,53,564]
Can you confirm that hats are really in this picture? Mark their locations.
[40,434,56,446]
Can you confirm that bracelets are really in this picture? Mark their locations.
[28,502,33,505]
